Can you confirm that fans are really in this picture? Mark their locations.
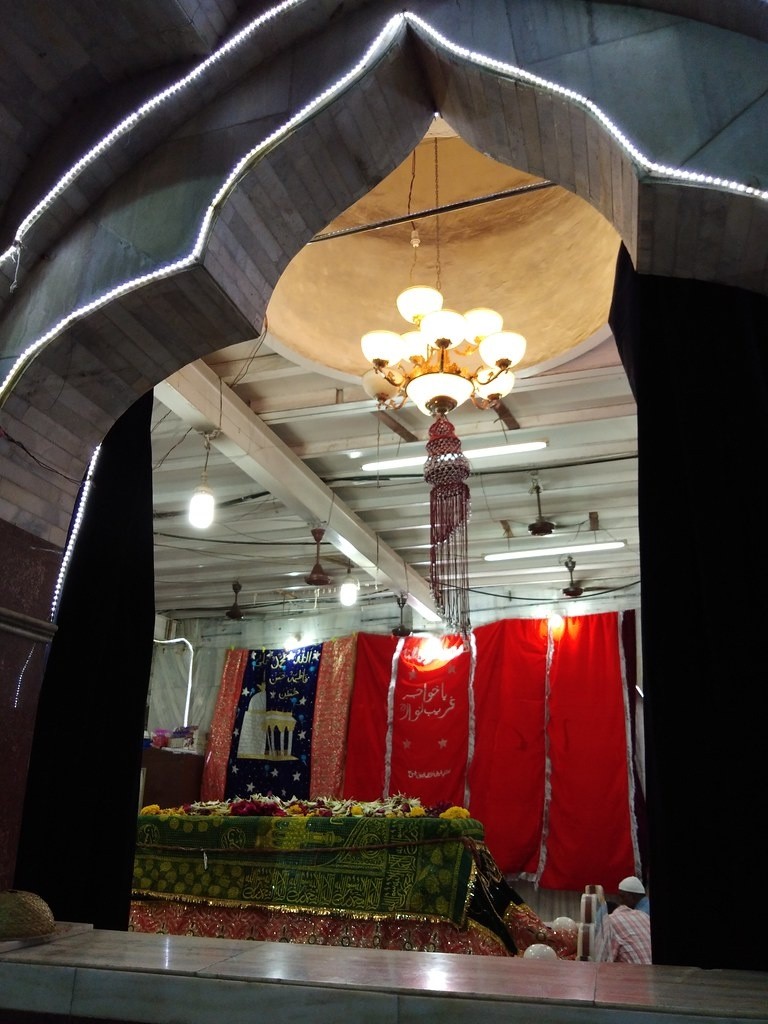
[225,582,245,620]
[304,528,331,586]
[562,556,615,598]
[391,597,428,637]
[527,485,568,536]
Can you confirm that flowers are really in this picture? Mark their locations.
[141,792,471,820]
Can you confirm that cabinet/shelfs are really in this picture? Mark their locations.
[141,746,204,809]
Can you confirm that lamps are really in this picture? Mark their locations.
[188,445,215,529]
[359,136,528,639]
[339,559,360,607]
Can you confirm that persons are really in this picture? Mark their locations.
[592,901,652,964]
[619,875,649,915]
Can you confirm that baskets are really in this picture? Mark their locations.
[0,889,54,938]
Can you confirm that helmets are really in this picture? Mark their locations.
[619,876,646,893]
